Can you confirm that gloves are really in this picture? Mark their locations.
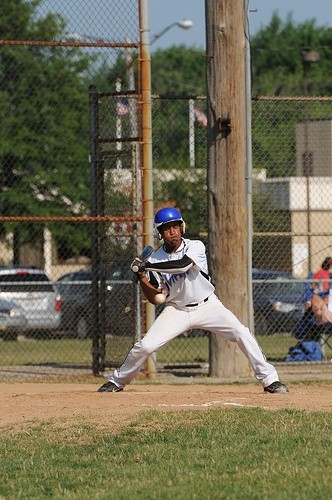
[130,256,151,279]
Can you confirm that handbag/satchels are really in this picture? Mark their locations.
[286,341,324,362]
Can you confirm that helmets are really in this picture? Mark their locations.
[153,207,185,240]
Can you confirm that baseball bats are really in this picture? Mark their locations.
[132,246,153,272]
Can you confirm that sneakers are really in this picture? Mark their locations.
[97,381,123,394]
[264,381,289,394]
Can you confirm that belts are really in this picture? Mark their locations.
[168,297,208,307]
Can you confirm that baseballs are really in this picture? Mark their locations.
[155,294,166,303]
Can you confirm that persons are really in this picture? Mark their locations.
[97,208,289,394]
[311,257,332,361]
